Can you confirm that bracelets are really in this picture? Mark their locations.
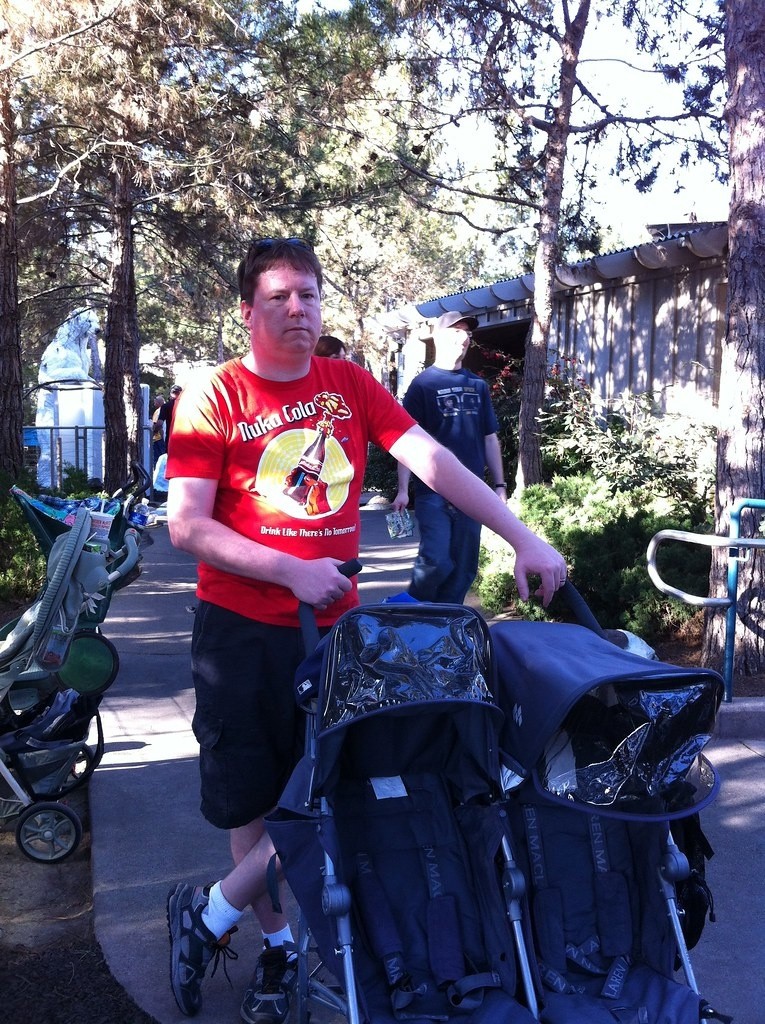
[494,482,507,489]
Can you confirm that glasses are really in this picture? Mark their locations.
[449,329,473,339]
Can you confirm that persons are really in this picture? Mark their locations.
[390,311,508,608]
[152,384,183,452]
[150,396,167,471]
[312,335,347,361]
[162,240,574,1023]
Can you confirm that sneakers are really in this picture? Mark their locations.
[166,880,241,1019]
[245,945,299,1023]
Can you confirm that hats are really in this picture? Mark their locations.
[434,310,479,330]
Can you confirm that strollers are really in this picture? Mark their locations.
[276,554,740,1023]
[0,459,152,862]
